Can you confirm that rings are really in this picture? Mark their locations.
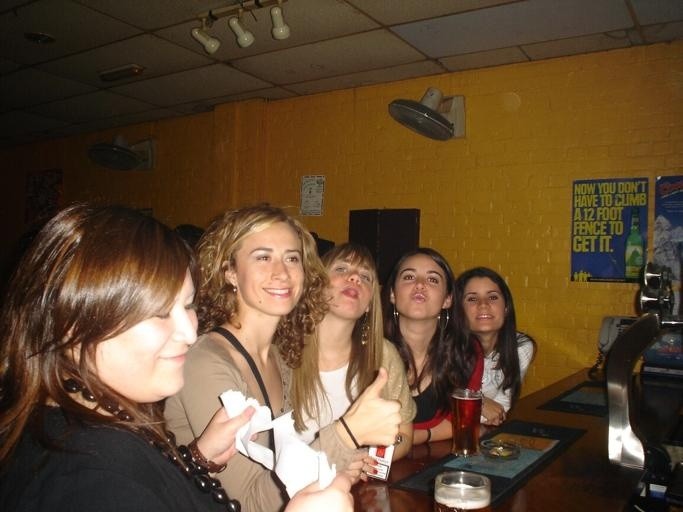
[499,415,506,422]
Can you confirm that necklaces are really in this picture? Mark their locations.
[64,378,244,507]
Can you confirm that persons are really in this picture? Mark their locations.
[165,203,402,507]
[289,241,417,489]
[0,197,356,507]
[453,267,534,427]
[380,248,485,446]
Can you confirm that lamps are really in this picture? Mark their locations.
[270,5,291,41]
[189,23,221,54]
[226,14,255,49]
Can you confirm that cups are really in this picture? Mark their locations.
[434,471,492,511]
[451,387,482,457]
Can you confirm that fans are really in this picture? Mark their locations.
[84,136,155,173]
[386,84,467,143]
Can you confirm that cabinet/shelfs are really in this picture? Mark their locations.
[348,365,683,512]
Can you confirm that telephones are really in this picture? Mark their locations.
[588,316,641,374]
